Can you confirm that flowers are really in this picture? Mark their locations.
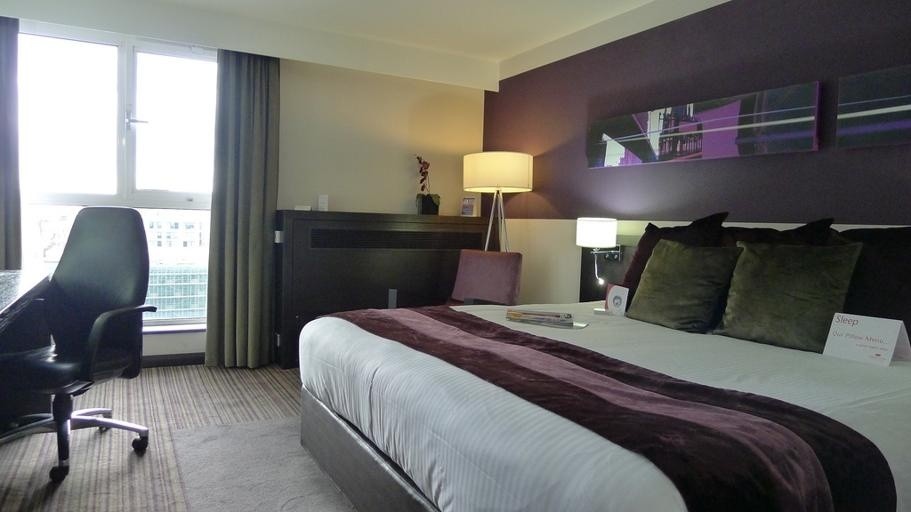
[416,153,432,193]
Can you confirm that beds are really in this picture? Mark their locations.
[299,223,911,512]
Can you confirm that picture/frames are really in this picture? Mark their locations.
[461,196,477,216]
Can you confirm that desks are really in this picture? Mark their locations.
[0,269,51,412]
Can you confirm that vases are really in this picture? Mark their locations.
[417,194,439,215]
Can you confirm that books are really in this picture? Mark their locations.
[505,308,589,330]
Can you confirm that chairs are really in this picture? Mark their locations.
[450,251,520,305]
[0,206,157,476]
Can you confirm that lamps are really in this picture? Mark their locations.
[462,152,533,250]
[575,217,619,284]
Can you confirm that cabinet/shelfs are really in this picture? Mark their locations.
[272,209,498,369]
